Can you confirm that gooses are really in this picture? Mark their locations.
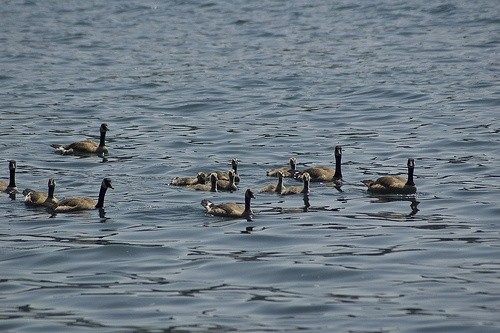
[302,142,344,183]
[22,176,59,208]
[49,123,111,156]
[0,159,20,194]
[201,188,257,219]
[266,157,300,179]
[254,169,286,197]
[359,156,418,195]
[171,157,240,193]
[279,172,312,196]
[54,178,115,212]
